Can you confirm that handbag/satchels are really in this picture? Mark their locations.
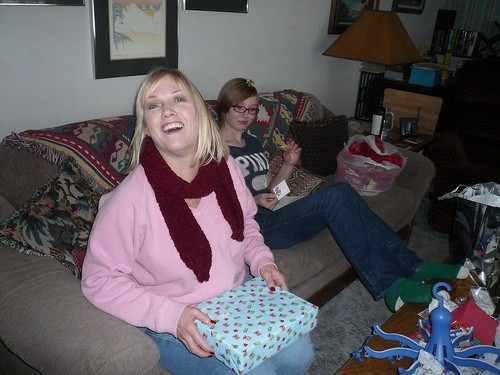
[436,180,500,289]
[292,115,350,176]
[334,134,404,196]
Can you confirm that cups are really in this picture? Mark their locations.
[399,117,418,138]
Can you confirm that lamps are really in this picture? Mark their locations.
[384,75,386,76]
[323,9,423,122]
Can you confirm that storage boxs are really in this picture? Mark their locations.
[408,67,443,87]
[409,297,497,348]
[194,278,318,375]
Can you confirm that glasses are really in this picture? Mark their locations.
[230,105,260,115]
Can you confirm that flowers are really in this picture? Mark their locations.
[246,79,255,86]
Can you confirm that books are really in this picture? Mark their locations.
[360,62,411,80]
[435,29,483,57]
[411,61,443,69]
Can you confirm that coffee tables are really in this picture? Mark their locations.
[332,277,471,375]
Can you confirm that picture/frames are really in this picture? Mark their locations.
[94,0,177,80]
[328,0,380,34]
[391,0,426,15]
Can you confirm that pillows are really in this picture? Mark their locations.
[291,115,348,177]
[0,155,108,281]
[269,152,327,198]
[0,89,437,375]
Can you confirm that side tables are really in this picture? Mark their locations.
[345,115,438,155]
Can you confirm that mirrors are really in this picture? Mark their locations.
[431,9,457,53]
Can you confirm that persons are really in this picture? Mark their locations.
[80,67,316,375]
[212,77,465,312]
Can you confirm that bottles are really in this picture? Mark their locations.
[441,66,448,85]
[370,104,385,140]
[381,106,392,141]
[424,50,431,63]
[441,48,452,65]
